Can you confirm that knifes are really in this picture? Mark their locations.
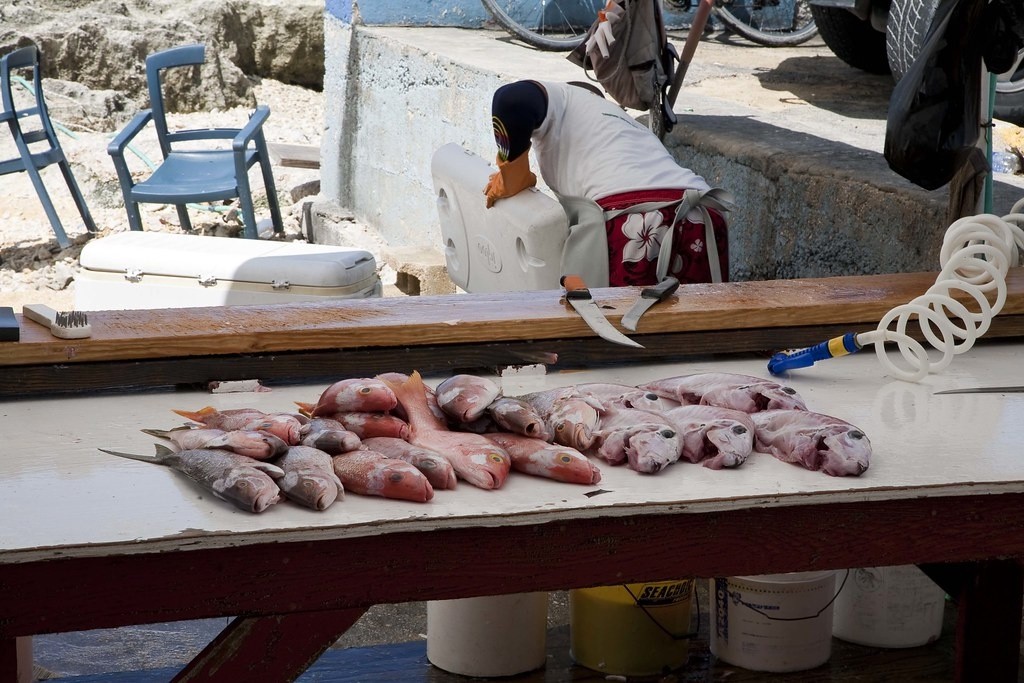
[620,275,679,331]
[559,274,646,349]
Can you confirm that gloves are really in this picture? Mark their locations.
[585,1,624,58]
[482,141,537,209]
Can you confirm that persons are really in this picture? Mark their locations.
[482,82,738,292]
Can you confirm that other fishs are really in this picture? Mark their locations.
[96,370,872,514]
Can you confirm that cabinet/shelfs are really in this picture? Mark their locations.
[0,270,1024,682]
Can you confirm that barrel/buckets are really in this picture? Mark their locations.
[832,566,946,649]
[569,580,701,676]
[708,568,848,672]
[427,591,549,677]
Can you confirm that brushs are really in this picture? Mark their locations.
[22,304,92,340]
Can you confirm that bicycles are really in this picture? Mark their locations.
[482,0,819,52]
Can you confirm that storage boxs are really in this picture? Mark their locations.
[431,142,569,294]
[75,231,382,311]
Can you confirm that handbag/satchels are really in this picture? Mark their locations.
[582,0,677,133]
[884,0,985,191]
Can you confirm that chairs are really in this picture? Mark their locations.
[0,44,98,249]
[107,44,284,240]
